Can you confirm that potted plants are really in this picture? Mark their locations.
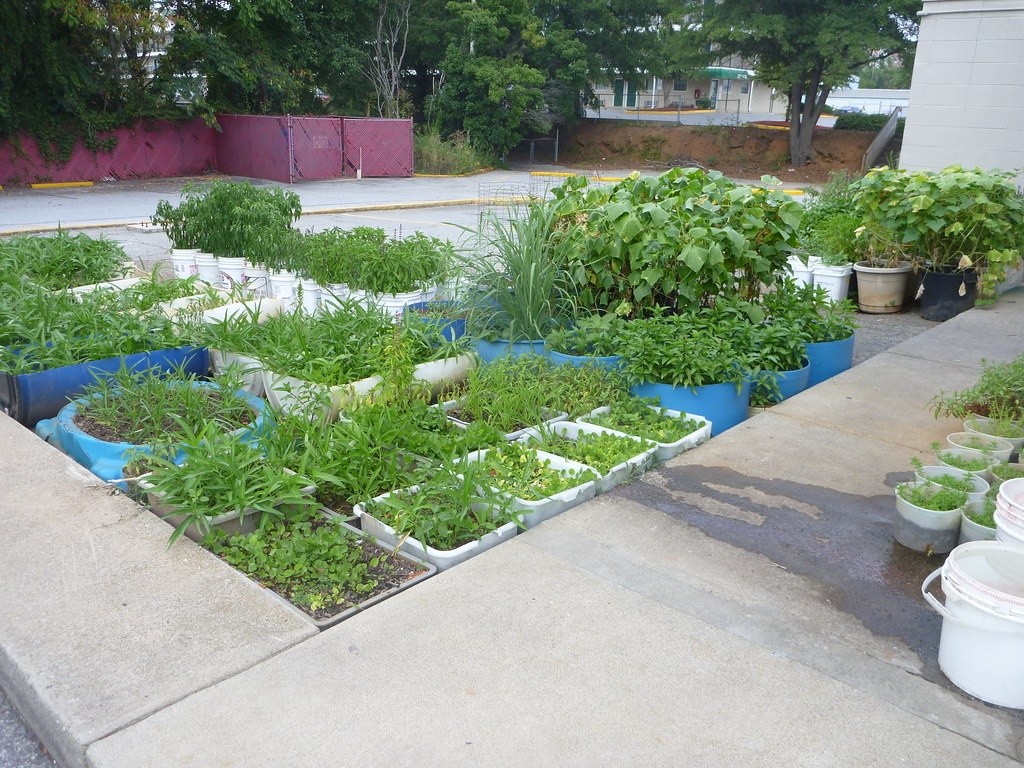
[0,162,1024,632]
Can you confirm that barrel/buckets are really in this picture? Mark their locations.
[921,540,1024,711]
[993,477,1024,540]
